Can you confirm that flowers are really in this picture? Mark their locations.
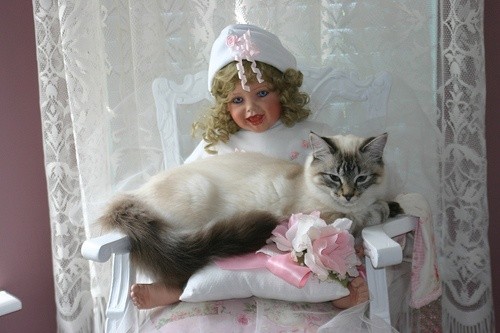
[266,211,361,281]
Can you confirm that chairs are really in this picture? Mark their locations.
[81,65,419,333]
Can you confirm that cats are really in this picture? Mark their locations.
[97,131,390,290]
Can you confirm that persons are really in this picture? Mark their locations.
[130,24,369,309]
